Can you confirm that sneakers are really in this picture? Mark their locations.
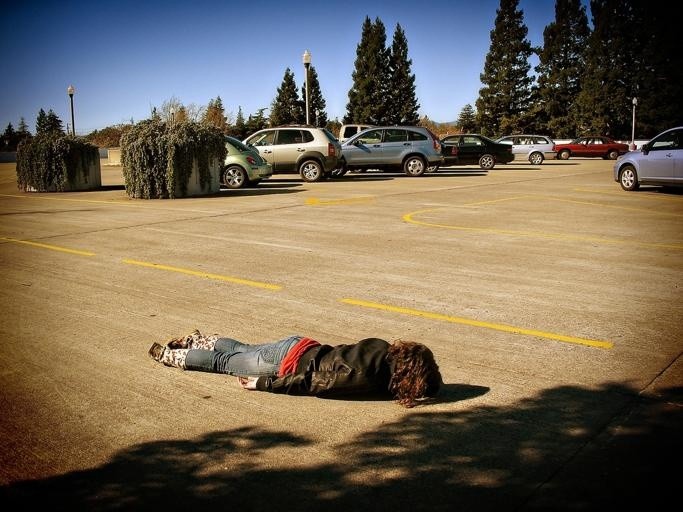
[167,329,200,348]
[148,342,165,359]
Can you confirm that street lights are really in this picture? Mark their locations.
[632,96,637,153]
[302,49,310,128]
[67,83,76,136]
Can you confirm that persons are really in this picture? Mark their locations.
[148,327,444,410]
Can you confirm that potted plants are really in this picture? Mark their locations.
[119,120,227,199]
[15,134,102,192]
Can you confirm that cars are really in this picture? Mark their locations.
[613,127,683,190]
[425,133,514,174]
[219,133,273,188]
[554,136,629,160]
[234,127,340,181]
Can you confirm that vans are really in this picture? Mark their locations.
[326,124,446,178]
[494,134,557,165]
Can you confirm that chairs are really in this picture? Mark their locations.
[385,134,407,141]
[446,139,464,155]
[512,139,536,145]
[584,139,595,145]
[273,135,312,145]
